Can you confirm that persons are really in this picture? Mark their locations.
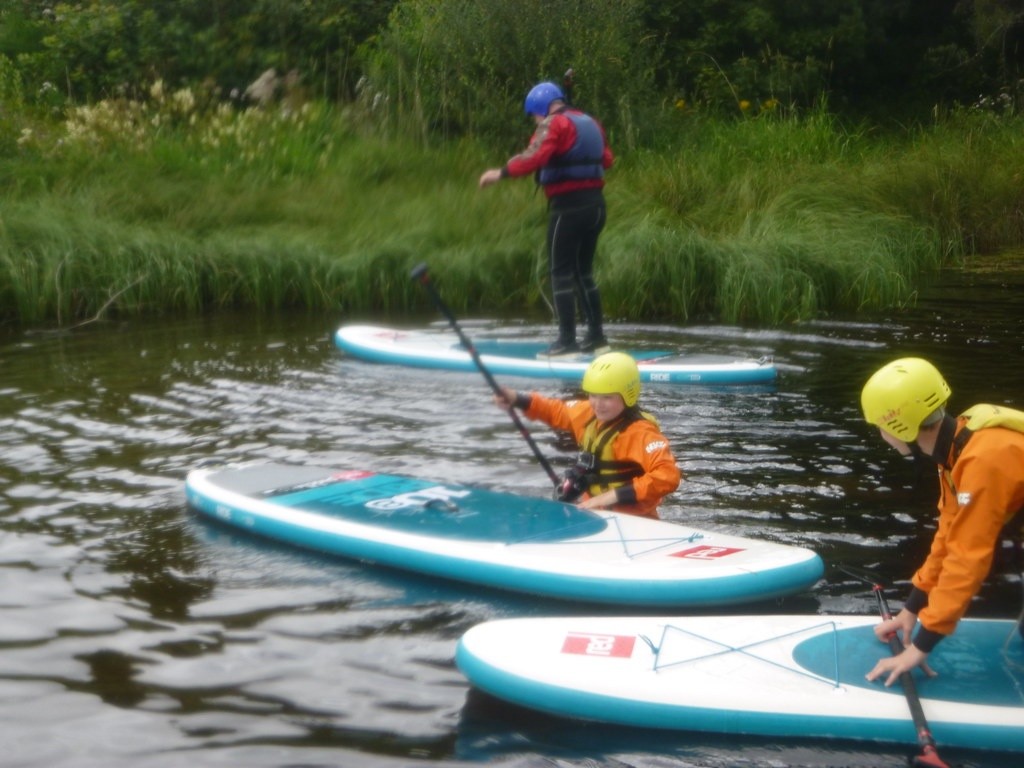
[493,352,681,521]
[479,83,613,362]
[861,358,1024,686]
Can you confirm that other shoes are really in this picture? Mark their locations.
[536,340,580,359]
[577,336,611,355]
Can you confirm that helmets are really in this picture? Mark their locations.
[861,357,952,445]
[524,82,567,118]
[582,352,641,408]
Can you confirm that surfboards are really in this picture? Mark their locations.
[452,613,1024,748]
[332,322,778,386]
[184,447,826,611]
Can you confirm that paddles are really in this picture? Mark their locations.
[874,568,956,768]
[406,259,577,501]
